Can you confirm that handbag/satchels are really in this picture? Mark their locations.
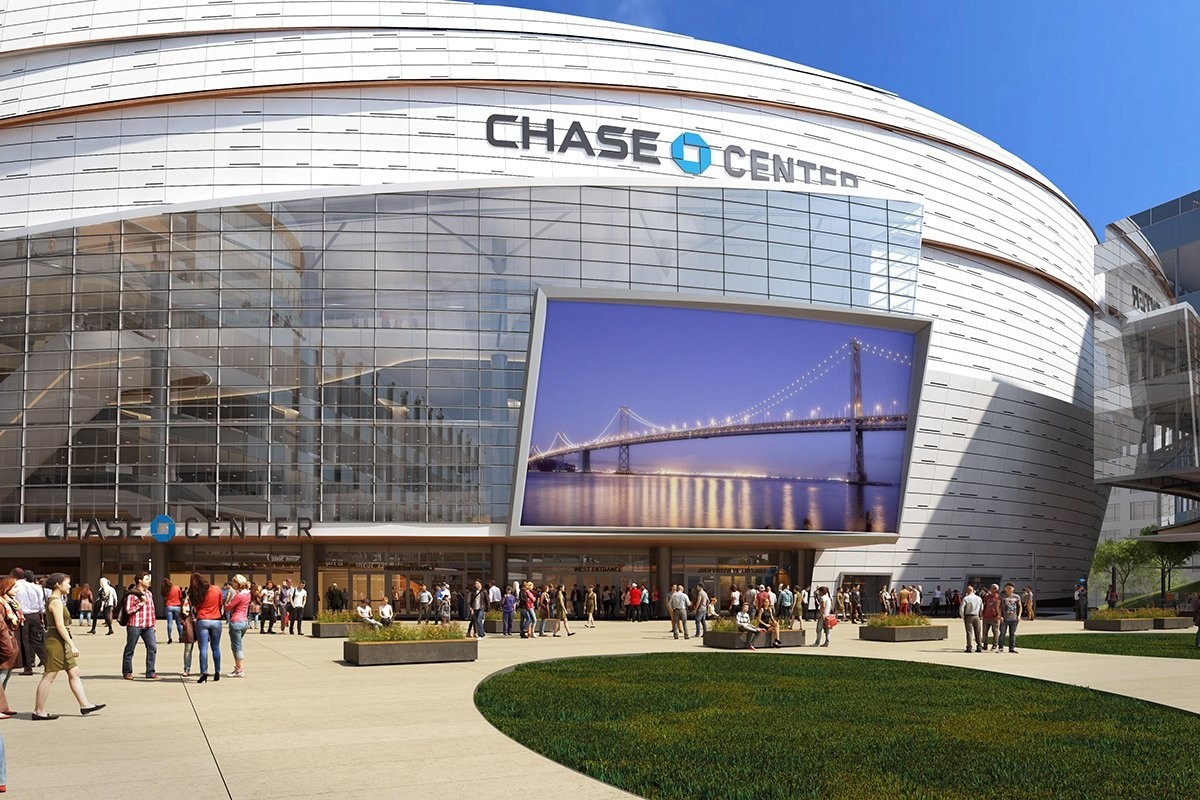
[824,615,837,629]
[116,606,130,626]
[286,603,292,612]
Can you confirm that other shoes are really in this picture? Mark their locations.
[250,627,304,635]
[757,628,766,632]
[0,714,11,719]
[775,638,782,644]
[32,712,59,721]
[670,630,704,639]
[2,711,17,714]
[748,645,755,650]
[80,703,106,715]
[787,618,867,630]
[183,671,189,677]
[124,674,133,679]
[773,643,781,648]
[466,625,596,640]
[228,666,245,677]
[106,629,114,636]
[809,643,819,647]
[168,638,172,644]
[820,643,828,647]
[19,661,43,675]
[964,646,1019,654]
[87,629,96,634]
[146,674,162,679]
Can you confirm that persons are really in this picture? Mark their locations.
[959,586,983,653]
[729,583,777,619]
[879,585,923,616]
[776,583,810,630]
[1165,591,1200,606]
[809,585,833,647]
[962,584,992,620]
[736,602,766,650]
[1104,585,1118,609]
[324,583,416,630]
[1073,584,1088,621]
[996,583,1022,654]
[0,566,307,719]
[0,735,7,793]
[414,579,658,641]
[757,598,782,648]
[665,582,720,639]
[980,584,1000,650]
[834,584,867,624]
[1021,585,1034,621]
[945,589,962,618]
[931,585,942,616]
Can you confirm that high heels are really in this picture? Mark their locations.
[197,674,208,683]
[214,672,220,680]
